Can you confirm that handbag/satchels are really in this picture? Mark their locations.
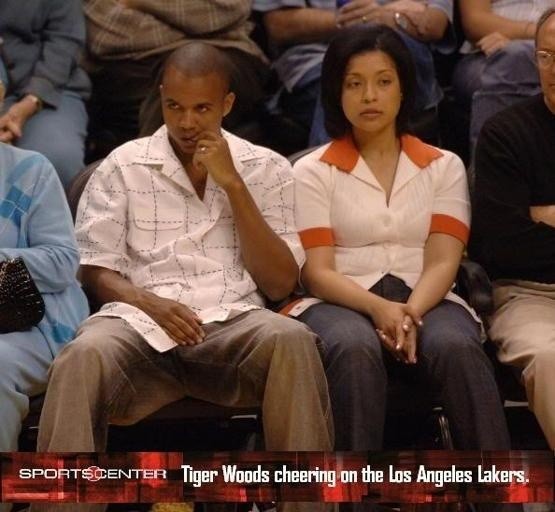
[1,256,45,333]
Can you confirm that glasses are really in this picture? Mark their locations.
[535,49,555,69]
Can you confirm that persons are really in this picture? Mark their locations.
[0,0,555,512]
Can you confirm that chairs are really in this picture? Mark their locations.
[68,160,284,512]
[287,146,470,512]
